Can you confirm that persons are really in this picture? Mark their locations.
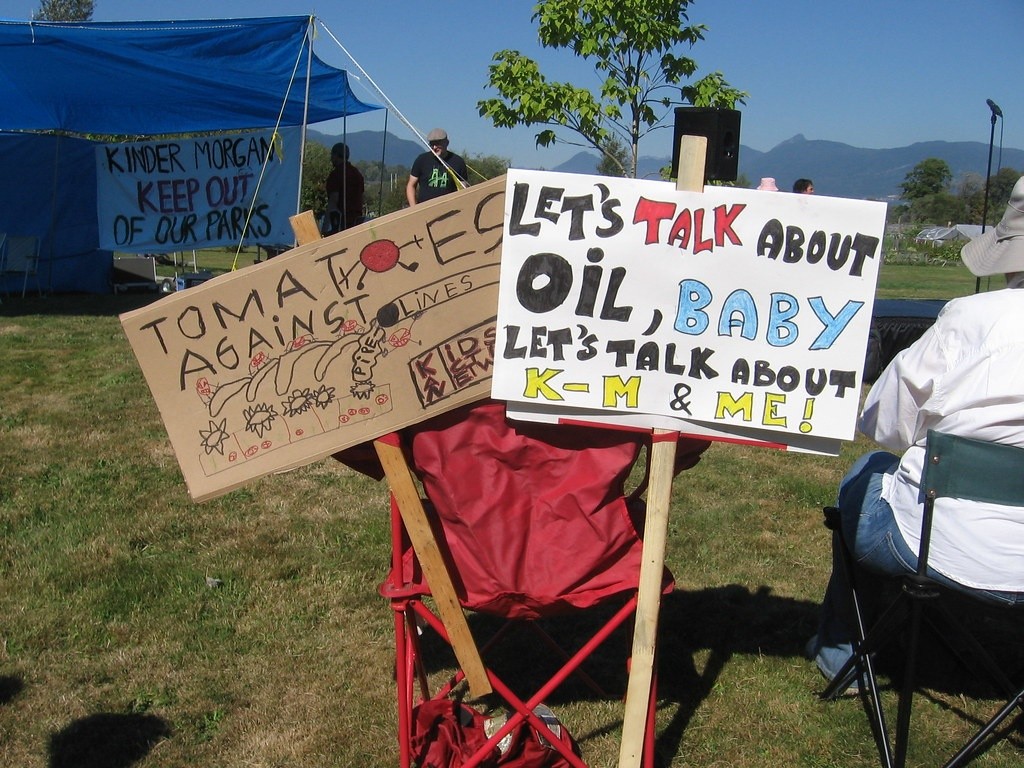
[407,128,468,206]
[794,179,814,195]
[322,142,364,238]
[807,174,1024,694]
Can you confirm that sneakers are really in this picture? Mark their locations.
[807,635,868,685]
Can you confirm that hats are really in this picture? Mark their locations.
[961,175,1024,277]
[427,128,447,141]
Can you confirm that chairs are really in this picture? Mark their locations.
[389,400,675,767]
[820,427,1024,768]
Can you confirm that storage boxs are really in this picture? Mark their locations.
[176,272,212,291]
[111,257,156,292]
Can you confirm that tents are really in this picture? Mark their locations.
[0,14,490,295]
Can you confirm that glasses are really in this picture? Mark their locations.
[428,141,448,146]
